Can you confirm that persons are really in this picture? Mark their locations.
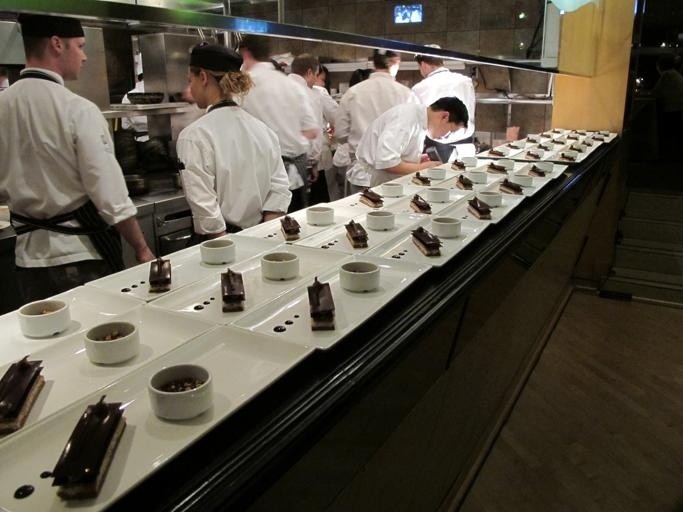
[121,73,149,157]
[270,45,476,208]
[233,35,320,213]
[0,20,157,305]
[175,44,292,247]
[647,53,682,161]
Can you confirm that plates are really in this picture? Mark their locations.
[295,211,431,254]
[0,304,217,442]
[0,284,147,371]
[147,242,352,327]
[330,156,568,225]
[0,324,317,512]
[363,214,490,266]
[231,252,433,351]
[85,232,283,303]
[474,128,617,161]
[237,202,366,242]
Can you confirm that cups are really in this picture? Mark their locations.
[16,298,70,340]
[260,251,299,280]
[198,238,235,266]
[339,262,379,292]
[147,362,216,421]
[84,320,138,366]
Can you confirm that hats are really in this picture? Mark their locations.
[16,13,84,37]
[190,44,243,76]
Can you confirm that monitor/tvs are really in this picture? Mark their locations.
[394,4,423,24]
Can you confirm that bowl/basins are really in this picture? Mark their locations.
[536,162,554,173]
[306,207,334,226]
[497,158,514,170]
[366,211,395,231]
[426,187,449,202]
[461,157,477,166]
[126,92,164,105]
[478,191,502,207]
[427,168,446,180]
[512,174,533,186]
[431,217,461,238]
[382,183,403,197]
[469,171,487,183]
[111,129,176,198]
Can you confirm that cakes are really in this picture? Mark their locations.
[306,275,336,330]
[342,124,610,257]
[148,255,171,292]
[0,353,46,431]
[280,213,300,241]
[52,394,128,500]
[220,267,245,312]
